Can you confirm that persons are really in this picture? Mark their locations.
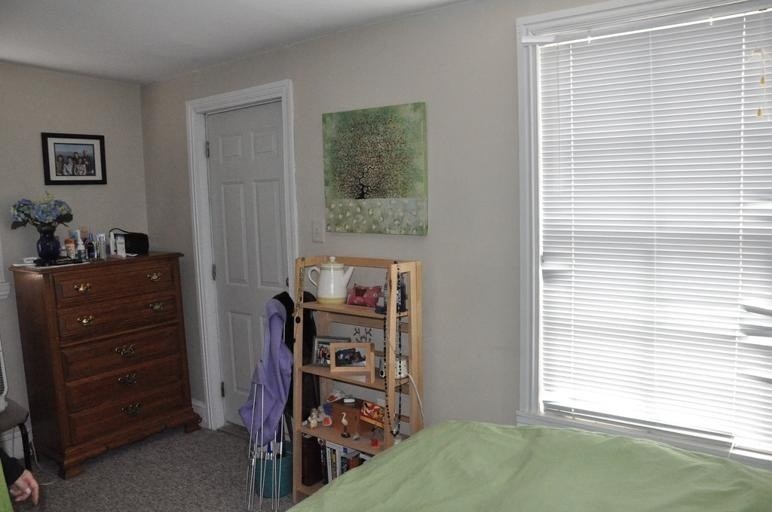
[0,445,40,511]
[56,151,90,176]
[318,346,329,368]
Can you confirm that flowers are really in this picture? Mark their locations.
[9,189,76,229]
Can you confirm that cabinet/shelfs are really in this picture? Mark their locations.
[11,249,202,480]
[289,253,425,506]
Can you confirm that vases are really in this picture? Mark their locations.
[32,222,62,264]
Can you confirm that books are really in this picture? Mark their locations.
[302,434,373,487]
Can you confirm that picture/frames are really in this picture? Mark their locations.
[39,132,110,186]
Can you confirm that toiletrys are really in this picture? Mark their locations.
[60,229,126,259]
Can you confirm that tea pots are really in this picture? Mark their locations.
[308,256,354,304]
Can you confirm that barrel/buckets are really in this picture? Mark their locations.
[248,441,292,498]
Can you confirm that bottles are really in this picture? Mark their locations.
[64,239,76,259]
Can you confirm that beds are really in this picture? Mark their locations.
[284,417,724,512]
